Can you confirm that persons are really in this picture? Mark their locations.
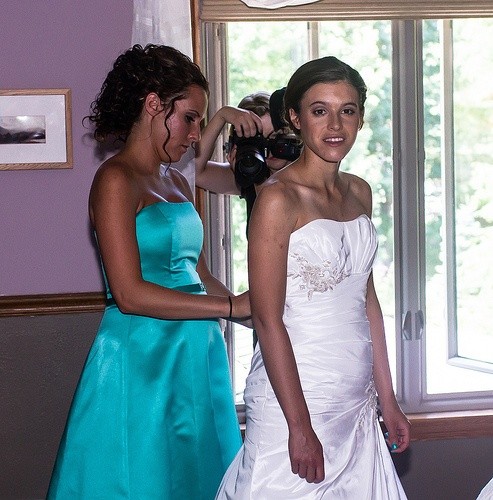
[195,92,293,241]
[213,56,413,500]
[46,45,253,500]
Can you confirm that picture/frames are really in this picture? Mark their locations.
[0,87,72,170]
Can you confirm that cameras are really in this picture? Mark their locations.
[228,124,302,187]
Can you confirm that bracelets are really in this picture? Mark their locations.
[226,295,234,320]
